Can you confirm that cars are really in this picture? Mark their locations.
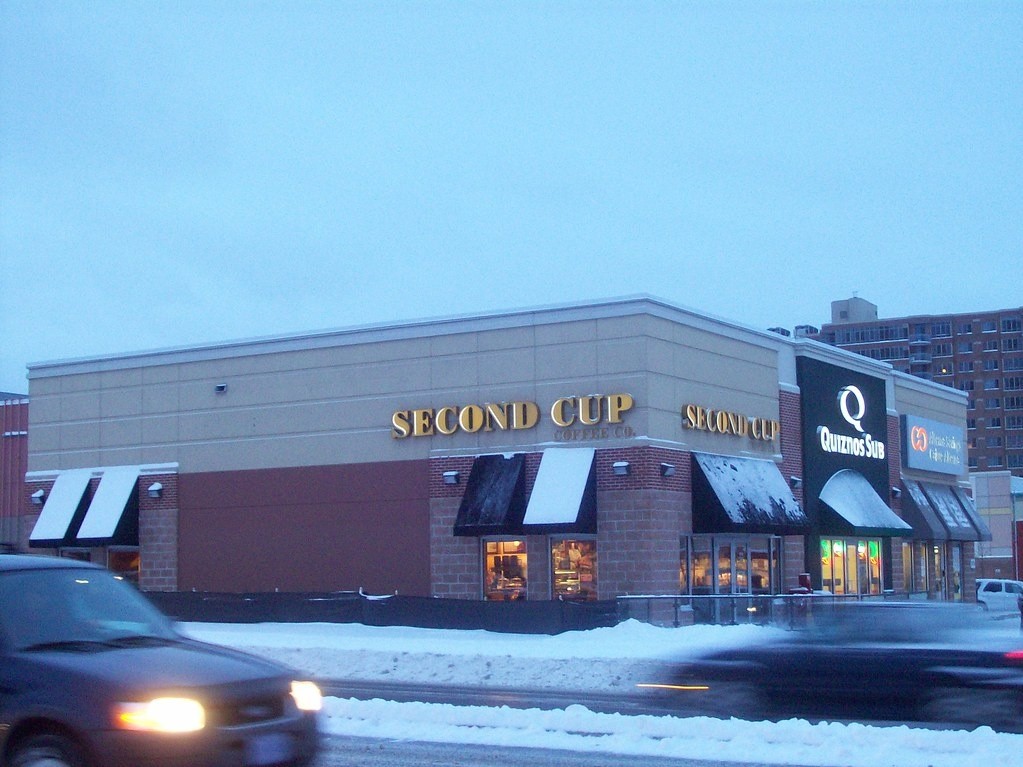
[975,578,1023,612]
[671,601,1023,730]
[0,552,325,767]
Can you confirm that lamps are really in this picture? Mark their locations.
[612,461,630,475]
[442,471,460,484]
[891,486,901,499]
[660,462,676,476]
[147,482,162,497]
[789,476,802,488]
[30,489,44,504]
